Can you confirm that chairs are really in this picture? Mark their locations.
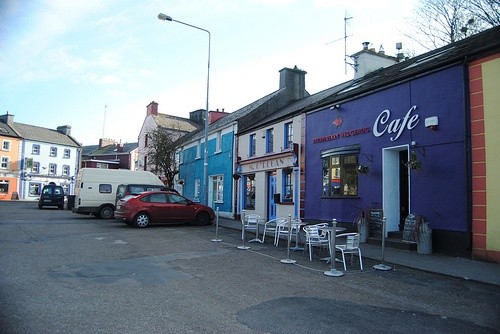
[335,232,363,271]
[276,219,332,261]
[245,214,261,238]
[262,217,287,245]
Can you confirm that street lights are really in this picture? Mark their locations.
[158,13,211,208]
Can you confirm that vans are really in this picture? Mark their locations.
[73,167,166,220]
[115,184,181,212]
[38,185,65,210]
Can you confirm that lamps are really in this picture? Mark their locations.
[412,141,425,157]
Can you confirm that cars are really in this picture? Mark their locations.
[114,191,216,228]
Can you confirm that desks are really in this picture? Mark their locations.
[318,226,346,264]
[286,222,310,252]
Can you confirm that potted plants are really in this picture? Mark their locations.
[284,165,293,173]
[405,149,423,170]
[360,164,368,173]
[247,175,254,180]
